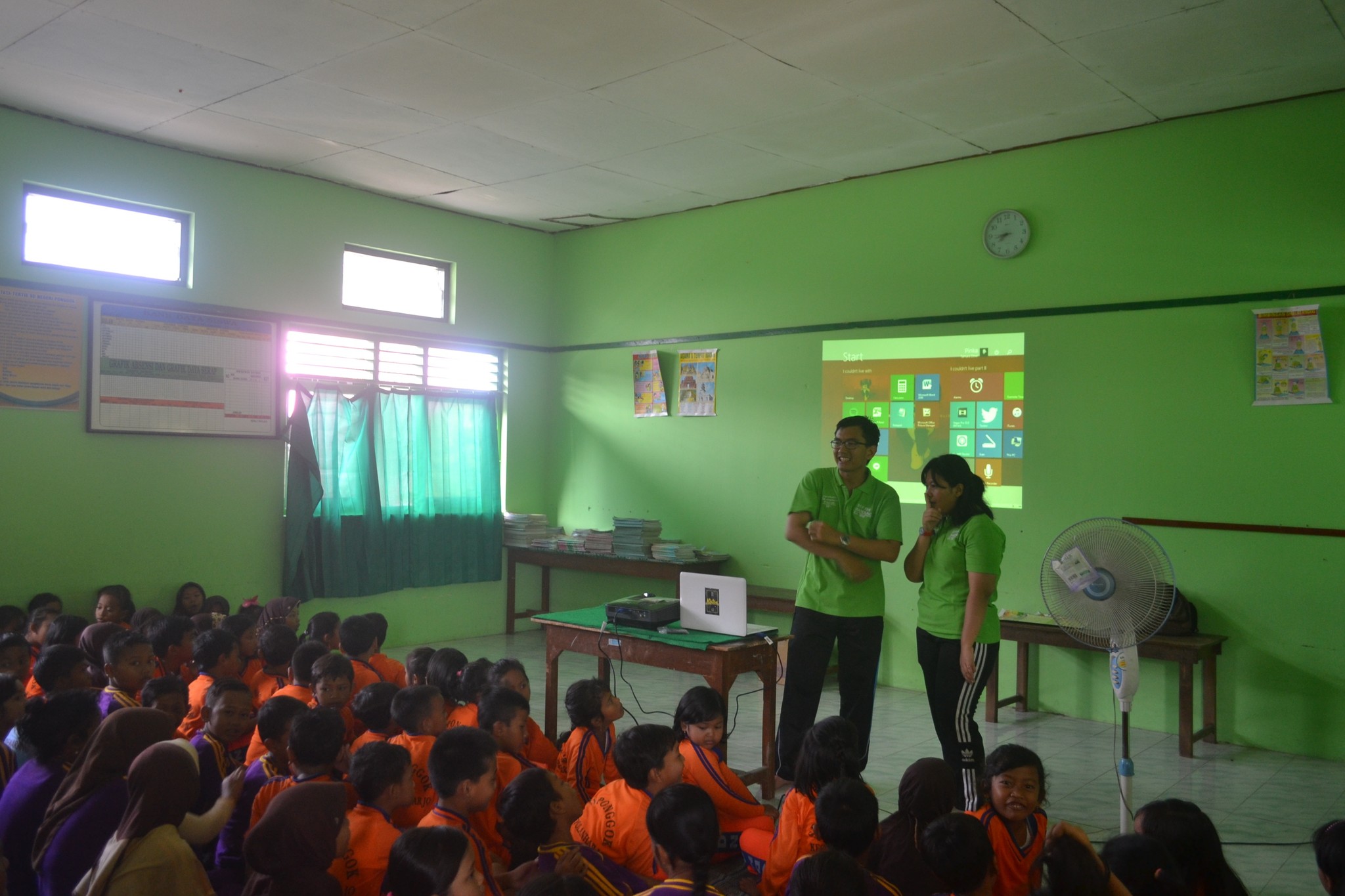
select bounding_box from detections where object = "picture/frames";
[83,297,282,439]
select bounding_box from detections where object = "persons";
[757,415,904,787]
[904,454,1006,809]
[0,578,1343,896]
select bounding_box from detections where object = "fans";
[1039,517,1176,842]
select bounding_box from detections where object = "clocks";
[982,209,1032,260]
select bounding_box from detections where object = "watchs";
[839,533,850,549]
[918,526,936,537]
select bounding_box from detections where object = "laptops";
[679,570,777,637]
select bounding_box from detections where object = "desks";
[747,584,797,617]
[524,605,779,812]
[502,545,728,636]
[982,608,1227,763]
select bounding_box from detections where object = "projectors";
[605,592,679,629]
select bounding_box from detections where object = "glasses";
[828,437,868,451]
